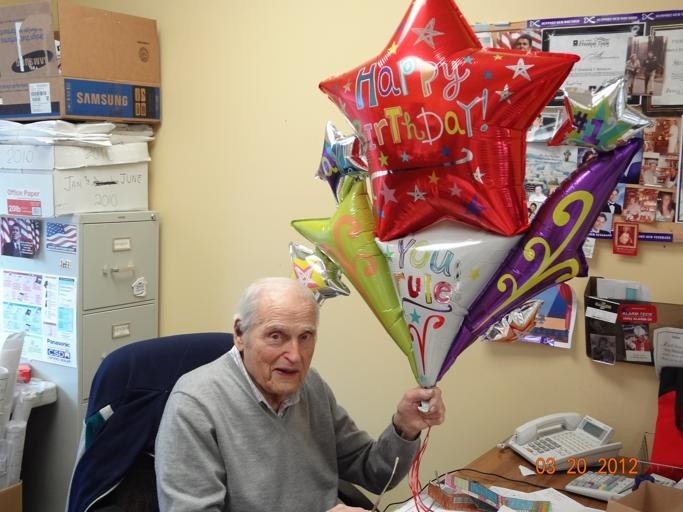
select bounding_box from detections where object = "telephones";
[495,412,624,472]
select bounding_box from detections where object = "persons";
[643,162,661,182]
[657,190,675,222]
[666,167,677,187]
[152,276,446,511]
[667,121,677,154]
[1,223,28,258]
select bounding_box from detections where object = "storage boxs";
[0,1,162,216]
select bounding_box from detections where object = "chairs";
[66,333,233,511]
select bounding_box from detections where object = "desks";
[395,435,681,512]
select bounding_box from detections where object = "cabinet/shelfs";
[1,211,159,512]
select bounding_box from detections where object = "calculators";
[564,471,636,502]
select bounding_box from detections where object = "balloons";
[286,0,655,413]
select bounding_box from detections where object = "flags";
[0,216,38,254]
[46,222,76,249]
